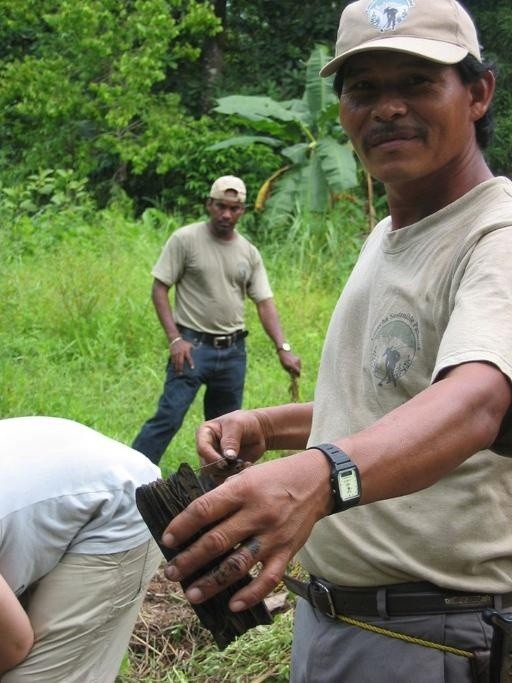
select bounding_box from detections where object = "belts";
[273,569,512,620]
[175,323,250,350]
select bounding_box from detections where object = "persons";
[131,173,301,466]
[166,0,510,680]
[0,413,164,682]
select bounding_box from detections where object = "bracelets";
[168,335,184,347]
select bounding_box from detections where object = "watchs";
[276,342,291,353]
[314,436,361,513]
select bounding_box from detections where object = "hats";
[316,0,484,79]
[208,174,247,203]
